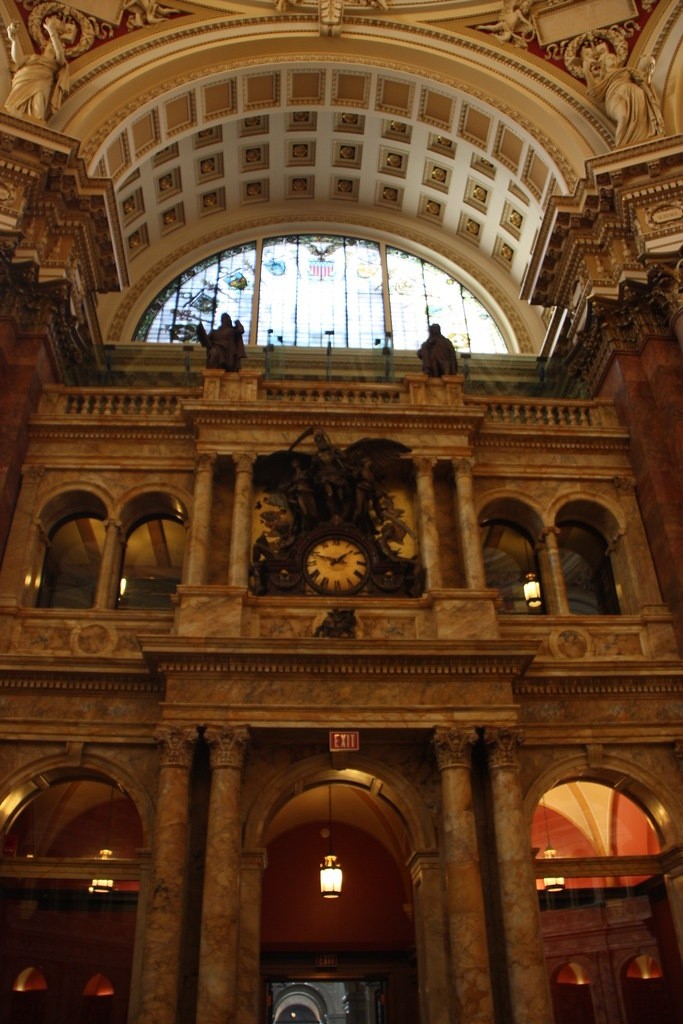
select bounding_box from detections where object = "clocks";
[304,534,373,597]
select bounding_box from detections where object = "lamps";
[91,786,118,893]
[522,542,542,610]
[319,784,343,898]
[540,796,566,892]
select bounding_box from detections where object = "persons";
[196,313,246,373]
[4,18,69,124]
[476,0,536,46]
[417,323,458,377]
[285,429,395,526]
[584,52,664,147]
